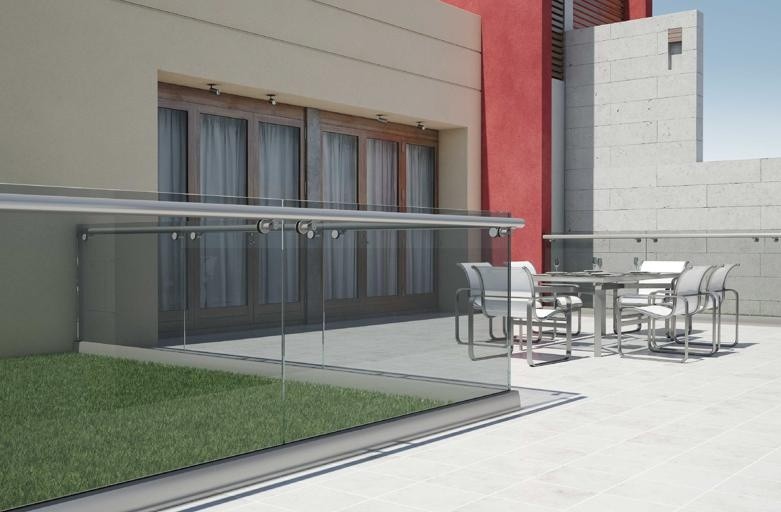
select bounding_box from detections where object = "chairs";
[653,259,742,357]
[454,259,543,350]
[615,262,719,364]
[468,260,577,366]
[501,259,585,341]
[614,257,690,340]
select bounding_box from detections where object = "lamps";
[206,84,220,96]
[376,113,389,125]
[415,121,427,133]
[266,93,277,107]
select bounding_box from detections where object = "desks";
[528,268,680,358]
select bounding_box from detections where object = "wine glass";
[552,254,560,272]
[591,256,603,270]
[634,257,645,272]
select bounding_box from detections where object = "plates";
[630,271,649,274]
[582,270,610,276]
[545,271,568,274]
